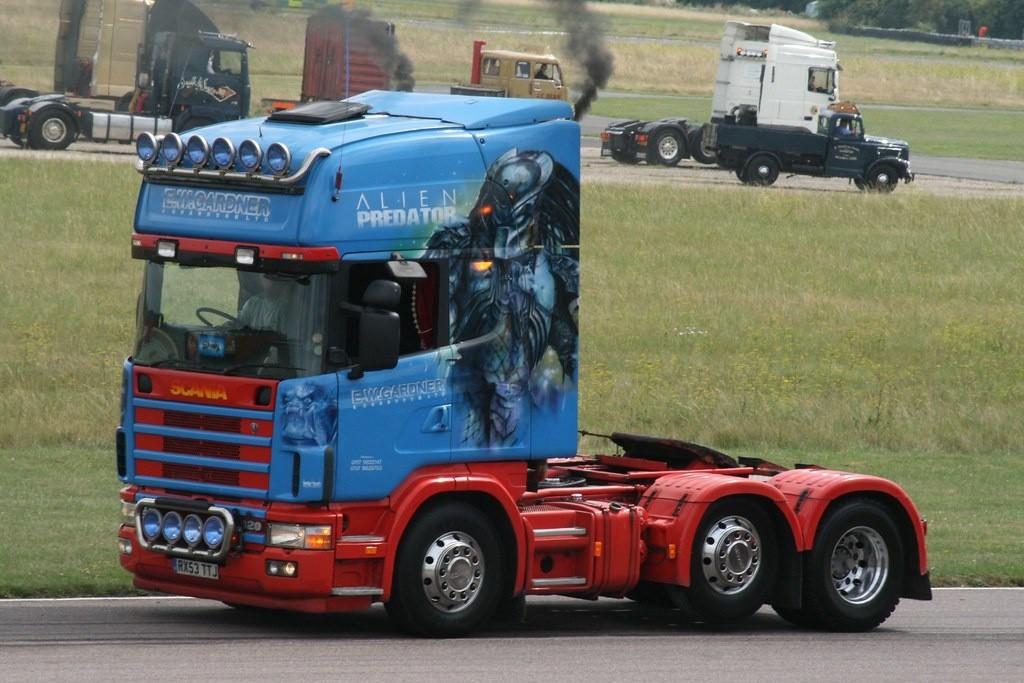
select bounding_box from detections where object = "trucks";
[261,6,395,112]
[451,40,568,104]
[0,29,257,151]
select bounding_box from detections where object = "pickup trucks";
[704,101,913,193]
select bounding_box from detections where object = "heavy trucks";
[601,19,843,168]
[115,90,934,641]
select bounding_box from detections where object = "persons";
[534,63,548,79]
[398,262,439,357]
[223,269,303,366]
[808,76,817,93]
